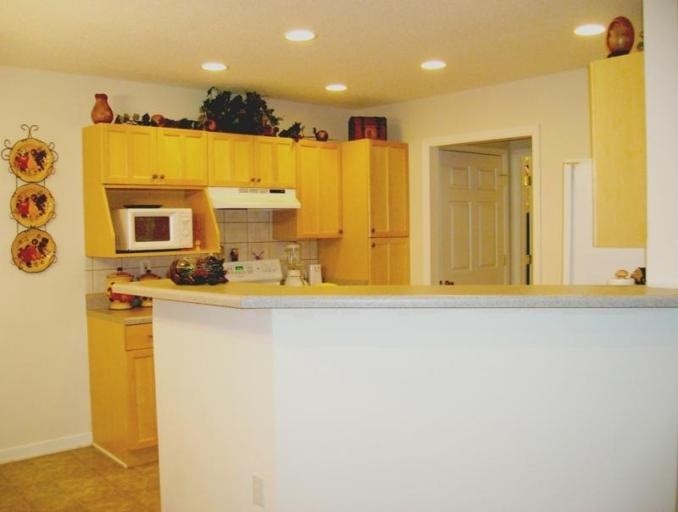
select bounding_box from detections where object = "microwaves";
[109,206,196,252]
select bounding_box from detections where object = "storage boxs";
[348,116,387,140]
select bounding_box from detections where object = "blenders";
[283,242,307,287]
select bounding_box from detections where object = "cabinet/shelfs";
[87,317,157,469]
[272,138,343,241]
[82,123,296,191]
[316,137,411,285]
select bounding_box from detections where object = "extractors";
[204,185,301,210]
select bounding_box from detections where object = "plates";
[10,137,57,273]
